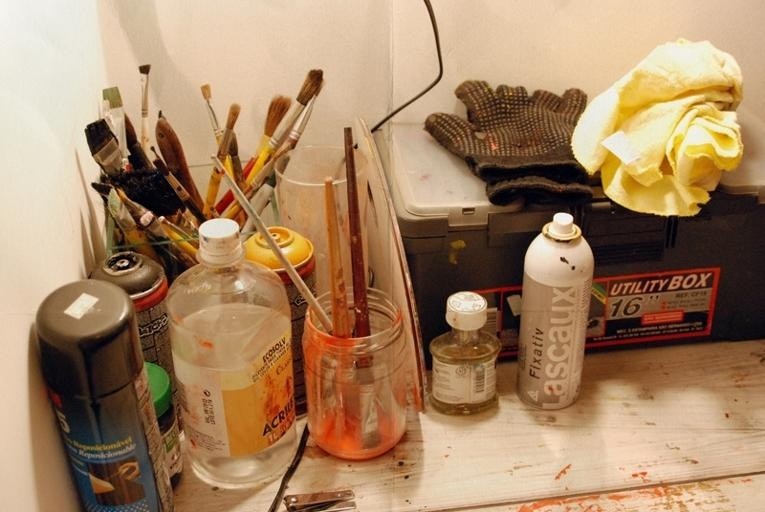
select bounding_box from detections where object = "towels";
[571,38,744,218]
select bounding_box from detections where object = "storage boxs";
[375,119,764,371]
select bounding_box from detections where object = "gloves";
[467,109,595,209]
[425,79,588,179]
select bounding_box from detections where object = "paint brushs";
[84,64,324,272]
[210,127,380,448]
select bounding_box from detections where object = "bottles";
[300,285,409,463]
[165,217,298,489]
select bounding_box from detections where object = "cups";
[272,143,370,297]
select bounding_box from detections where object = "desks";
[169,339,764,512]
